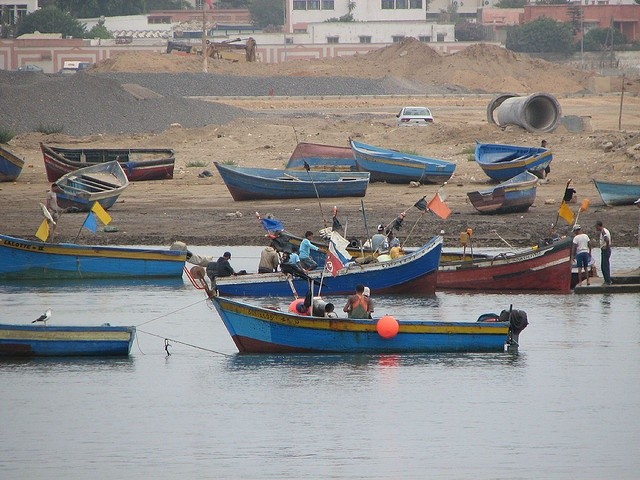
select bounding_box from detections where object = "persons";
[299,231,327,271]
[290,246,307,272]
[570,224,591,286]
[541,140,550,177]
[45,183,59,242]
[593,221,613,285]
[258,242,278,273]
[362,287,374,319]
[563,187,576,203]
[343,284,370,319]
[372,224,389,251]
[217,252,236,277]
[386,230,400,248]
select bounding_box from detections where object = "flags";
[558,201,574,224]
[329,230,353,267]
[261,218,284,231]
[428,194,451,219]
[581,198,589,211]
[83,212,97,234]
[34,219,50,241]
[91,200,112,225]
[414,196,428,211]
[325,251,342,277]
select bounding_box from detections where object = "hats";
[224,252,231,260]
[571,224,580,232]
[378,225,384,231]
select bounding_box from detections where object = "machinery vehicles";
[166,36,257,63]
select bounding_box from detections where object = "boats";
[592,177,640,207]
[204,284,527,355]
[467,169,539,214]
[0,146,24,182]
[347,136,456,187]
[39,142,176,181]
[208,229,446,298]
[0,325,136,357]
[285,140,356,169]
[51,156,130,212]
[213,162,370,201]
[0,234,188,280]
[474,137,552,182]
[255,211,595,273]
[437,236,575,290]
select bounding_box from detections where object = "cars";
[14,64,43,72]
[396,106,435,126]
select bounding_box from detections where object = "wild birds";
[31,308,54,326]
